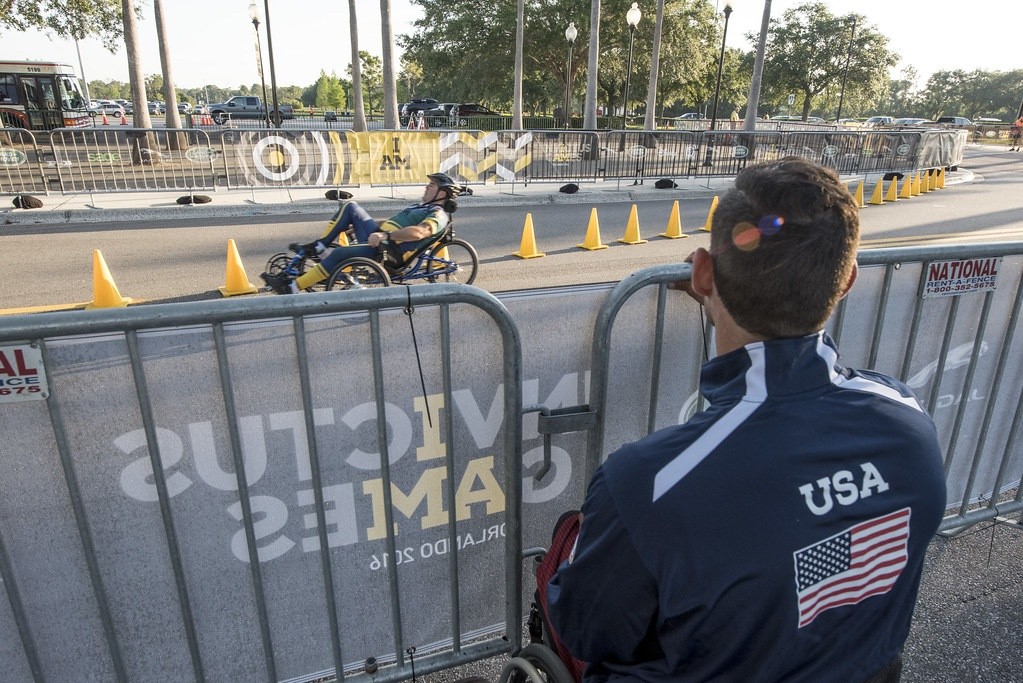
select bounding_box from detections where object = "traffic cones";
[513,212,546,260]
[100,110,129,126]
[218,239,258,297]
[854,165,946,208]
[335,231,366,284]
[421,241,450,269]
[617,206,649,244]
[577,208,609,251]
[189,111,213,125]
[84,249,131,310]
[659,193,724,239]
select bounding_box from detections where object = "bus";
[0,60,94,144]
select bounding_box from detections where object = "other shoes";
[261,272,291,294]
[1016,148,1020,151]
[1009,148,1014,151]
[288,242,319,257]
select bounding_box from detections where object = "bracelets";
[386,232,392,241]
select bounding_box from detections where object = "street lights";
[248,2,275,148]
[560,21,578,146]
[617,1,641,150]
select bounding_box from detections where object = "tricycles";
[265,198,479,325]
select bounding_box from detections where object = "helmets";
[427,173,461,199]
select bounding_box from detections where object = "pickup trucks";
[205,95,295,125]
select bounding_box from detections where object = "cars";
[675,112,1004,140]
[324,98,503,128]
[85,97,214,118]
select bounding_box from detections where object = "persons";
[310,108,314,119]
[260,172,463,295]
[1009,115,1023,151]
[546,154,947,683]
[730,106,740,130]
[763,112,769,119]
